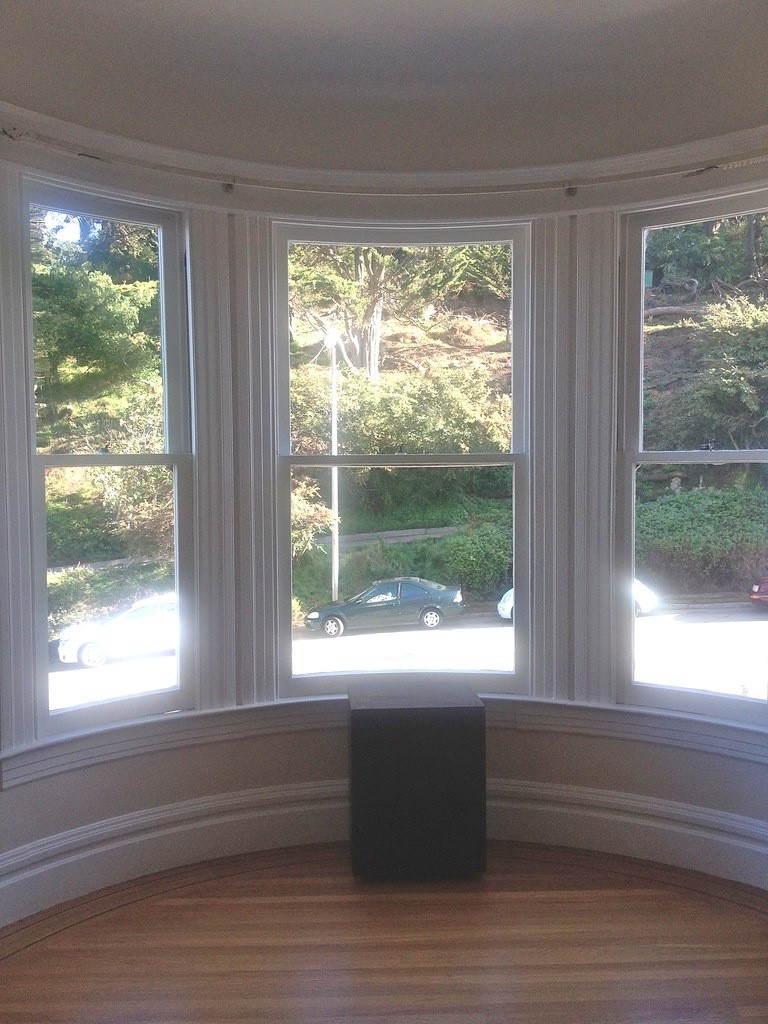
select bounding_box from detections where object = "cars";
[304,577,465,637]
[58,593,179,668]
[750,577,768,607]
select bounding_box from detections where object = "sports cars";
[498,576,662,620]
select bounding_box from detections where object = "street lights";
[322,331,343,602]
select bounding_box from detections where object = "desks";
[349,680,487,888]
